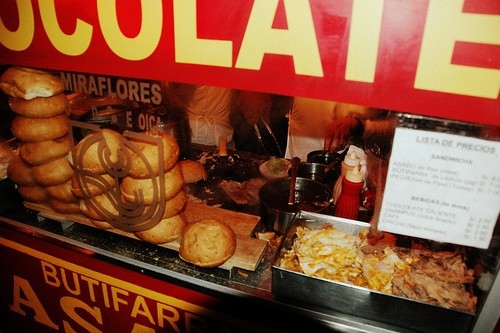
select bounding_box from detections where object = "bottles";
[332,151,360,206]
[333,164,365,219]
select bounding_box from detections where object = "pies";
[218,176,268,204]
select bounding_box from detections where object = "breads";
[0,66,236,268]
[259,157,292,180]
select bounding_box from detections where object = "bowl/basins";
[258,177,332,236]
[308,149,342,167]
[288,162,340,185]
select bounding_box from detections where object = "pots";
[61,88,190,163]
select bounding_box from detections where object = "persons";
[166,82,271,150]
[284,97,399,163]
